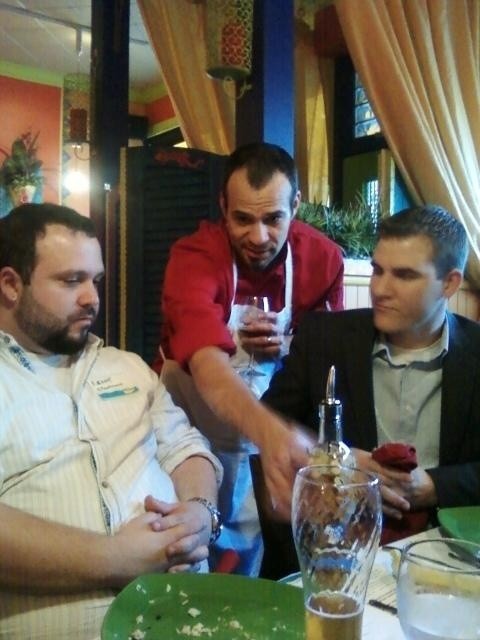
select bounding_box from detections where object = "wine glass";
[236,295,271,375]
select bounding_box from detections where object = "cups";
[291,466,479,640]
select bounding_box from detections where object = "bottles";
[308,363,357,471]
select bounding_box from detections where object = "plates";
[100,572,307,640]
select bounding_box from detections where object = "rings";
[267,334,272,345]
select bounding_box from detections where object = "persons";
[152,141,344,579]
[249,204,480,583]
[1,201,224,640]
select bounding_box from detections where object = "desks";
[266,521,479,638]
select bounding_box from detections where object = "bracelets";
[187,497,223,547]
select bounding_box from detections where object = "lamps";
[203,21,261,104]
[62,105,99,164]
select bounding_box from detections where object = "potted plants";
[4,151,42,208]
